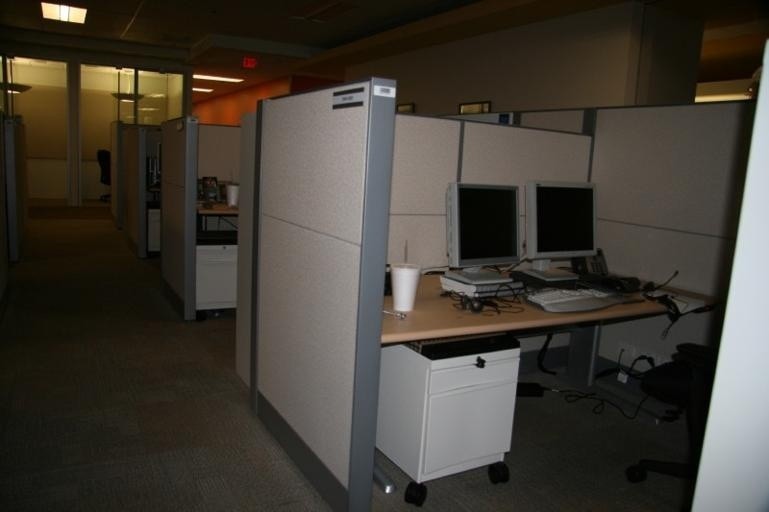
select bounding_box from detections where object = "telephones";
[573,247,609,276]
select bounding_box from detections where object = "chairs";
[625,358,718,511]
[96,150,112,203]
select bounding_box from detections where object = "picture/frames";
[395,102,415,113]
[459,101,491,115]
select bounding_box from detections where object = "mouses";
[643,290,669,300]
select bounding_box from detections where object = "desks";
[373,266,725,494]
[147,185,161,202]
[196,205,239,230]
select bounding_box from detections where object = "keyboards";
[524,286,628,312]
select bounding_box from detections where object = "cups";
[389,262,421,313]
[224,184,240,208]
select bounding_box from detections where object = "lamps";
[0,56,32,94]
[112,67,144,104]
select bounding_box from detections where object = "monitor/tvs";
[444,181,521,285]
[523,178,598,282]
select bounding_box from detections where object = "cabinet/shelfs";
[147,201,160,252]
[374,333,522,485]
[196,230,239,310]
